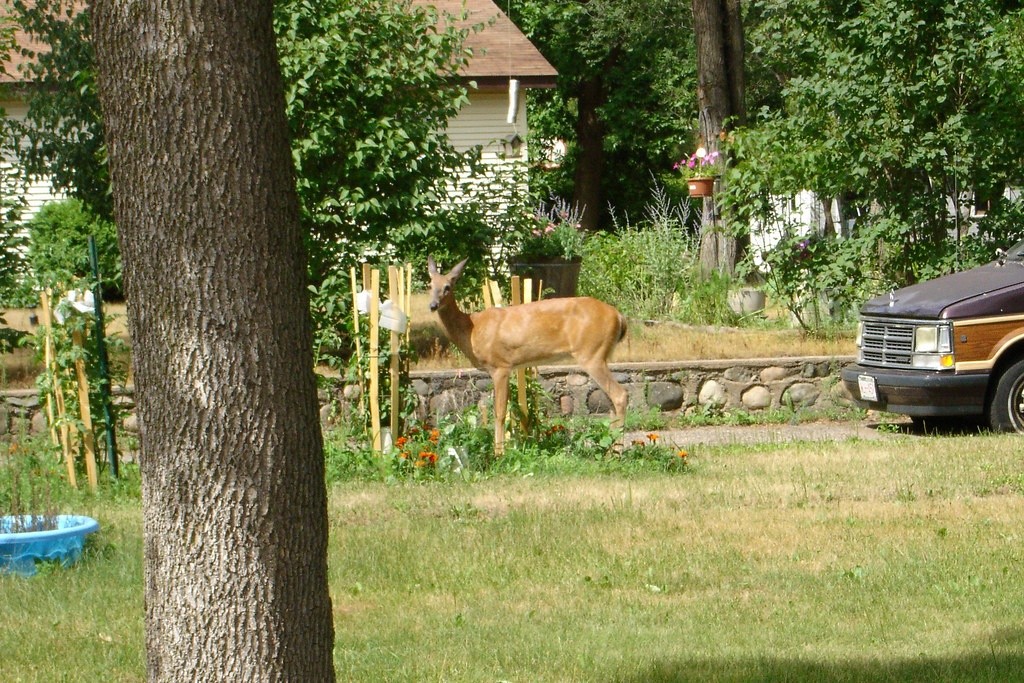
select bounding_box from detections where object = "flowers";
[673,148,723,180]
[508,207,585,263]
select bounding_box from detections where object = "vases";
[686,177,714,198]
[505,254,582,302]
[727,288,766,317]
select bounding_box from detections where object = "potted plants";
[0,409,99,575]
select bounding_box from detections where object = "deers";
[426,252,628,459]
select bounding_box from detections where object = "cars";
[840,237,1024,434]
